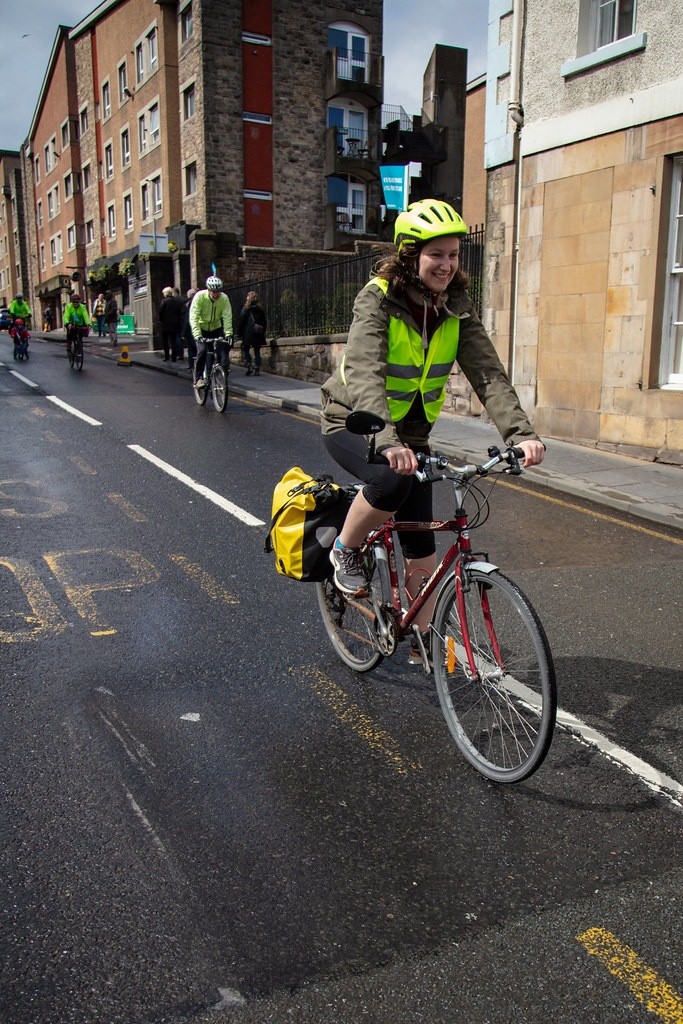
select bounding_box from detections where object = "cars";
[0,309,11,330]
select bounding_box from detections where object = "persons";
[44,303,54,332]
[10,293,32,348]
[92,290,118,347]
[188,276,234,399]
[11,319,30,360]
[320,199,544,682]
[64,293,92,361]
[238,291,268,376]
[159,286,201,369]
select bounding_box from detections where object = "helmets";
[394,199,469,254]
[15,293,23,299]
[70,294,80,300]
[15,319,24,325]
[206,276,223,291]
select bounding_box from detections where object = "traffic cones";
[117,345,133,366]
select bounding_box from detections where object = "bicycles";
[312,412,559,787]
[192,337,232,413]
[67,321,90,371]
[9,314,30,338]
[13,335,29,360]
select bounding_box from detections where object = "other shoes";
[254,368,259,376]
[246,367,252,377]
[162,354,195,369]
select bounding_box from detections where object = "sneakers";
[330,537,374,595]
[196,379,206,388]
[408,639,435,668]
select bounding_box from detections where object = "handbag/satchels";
[265,467,350,582]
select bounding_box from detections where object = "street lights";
[66,266,88,314]
